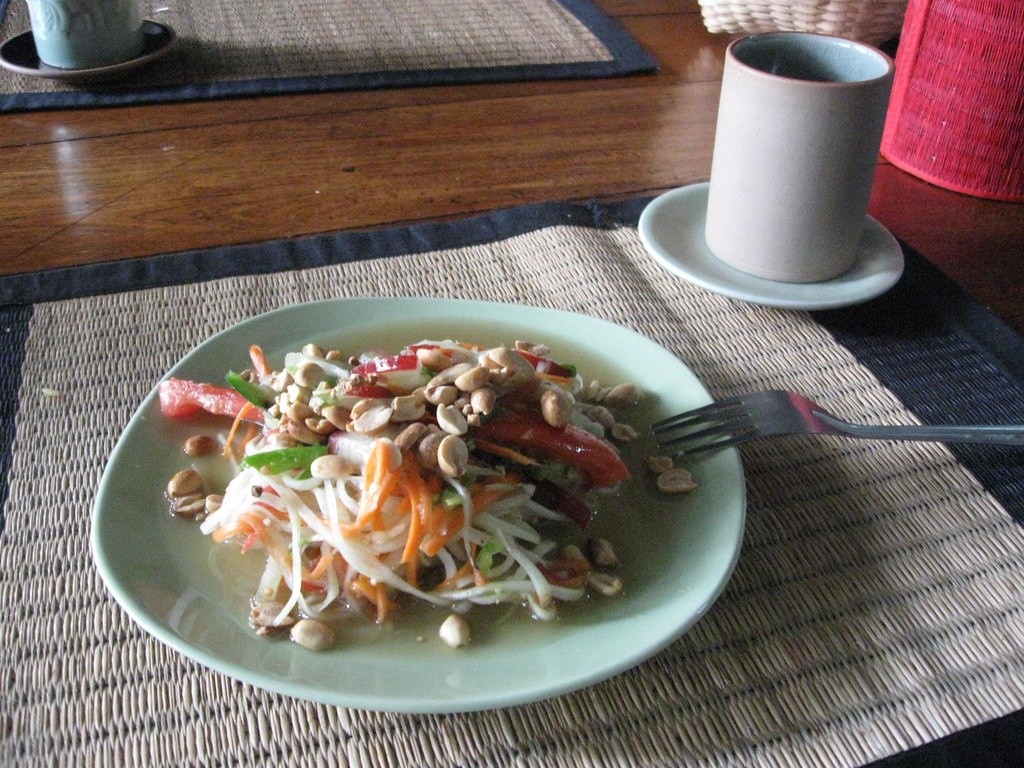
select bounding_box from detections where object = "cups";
[705,33,895,283]
[26,0,145,69]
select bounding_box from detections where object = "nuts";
[166,344,696,651]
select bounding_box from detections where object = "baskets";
[698,0,910,49]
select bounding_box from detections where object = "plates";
[89,298,749,715]
[0,19,176,83]
[637,181,903,310]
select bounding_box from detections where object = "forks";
[650,390,1023,461]
